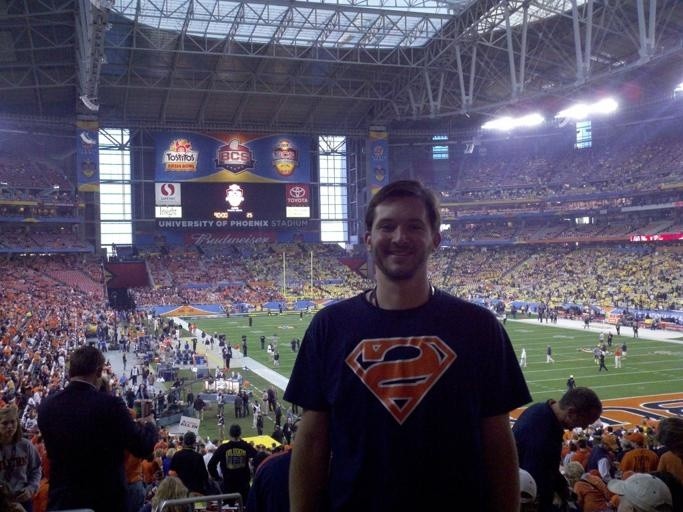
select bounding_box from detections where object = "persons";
[511,385,682,511]
[592,332,628,370]
[259,334,280,366]
[520,347,528,367]
[416,139,683,333]
[567,375,576,392]
[283,179,533,511]
[0,249,212,511]
[210,366,302,511]
[249,317,253,326]
[0,158,91,251]
[210,330,233,368]
[289,337,300,351]
[546,344,554,364]
[121,242,377,318]
[241,335,249,356]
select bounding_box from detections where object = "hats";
[625,434,643,444]
[608,472,673,511]
[518,469,537,502]
[605,435,618,449]
[184,432,196,444]
